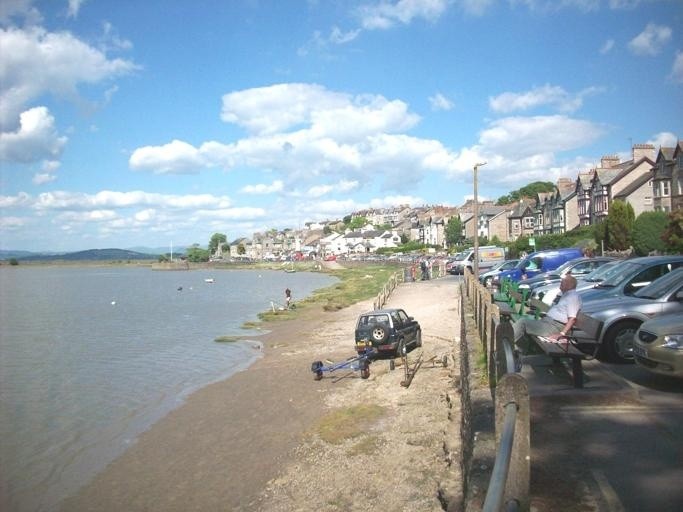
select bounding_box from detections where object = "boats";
[205,278,214,284]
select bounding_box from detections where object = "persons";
[286,288,291,301]
[511,270,583,344]
[584,248,592,257]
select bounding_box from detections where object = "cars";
[210,245,505,275]
[479,247,683,380]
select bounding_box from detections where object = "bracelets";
[560,331,566,336]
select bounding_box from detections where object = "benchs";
[487,277,604,390]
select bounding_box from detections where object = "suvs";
[352,309,422,358]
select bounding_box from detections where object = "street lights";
[472,160,489,283]
